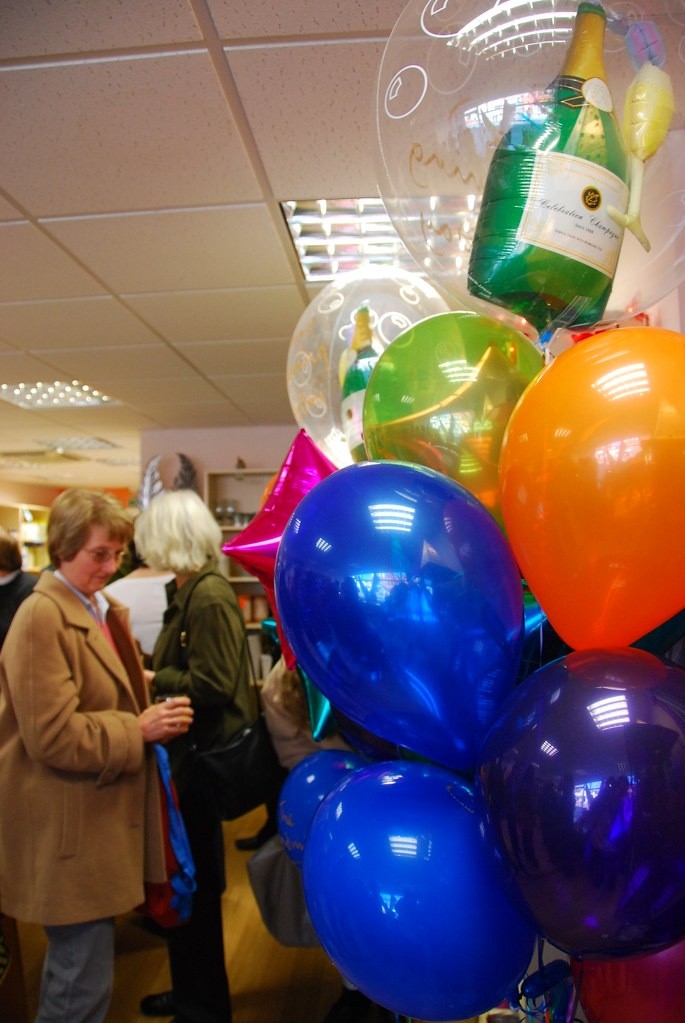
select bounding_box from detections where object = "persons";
[0,487,195,1023]
[0,527,40,651]
[133,488,406,1023]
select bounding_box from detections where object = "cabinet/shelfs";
[204,468,281,686]
[0,503,50,575]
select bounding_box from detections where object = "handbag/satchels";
[174,721,274,822]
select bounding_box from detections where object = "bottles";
[465,1,629,326]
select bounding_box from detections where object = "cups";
[156,695,188,729]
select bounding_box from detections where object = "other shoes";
[233,803,279,850]
[141,990,180,1017]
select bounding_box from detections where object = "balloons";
[220,429,338,670]
[277,750,538,1023]
[362,310,543,526]
[478,646,685,961]
[498,327,685,651]
[274,460,524,770]
[286,264,467,470]
[376,0,685,368]
[568,942,685,1023]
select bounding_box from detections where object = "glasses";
[78,545,127,562]
